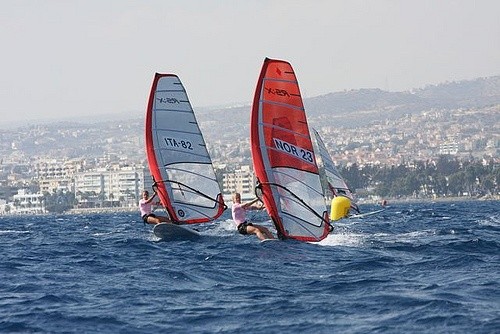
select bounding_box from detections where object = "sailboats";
[315,127,385,220]
[250,57,332,242]
[145,72,226,240]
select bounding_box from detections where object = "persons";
[139,190,171,225]
[232,193,275,240]
